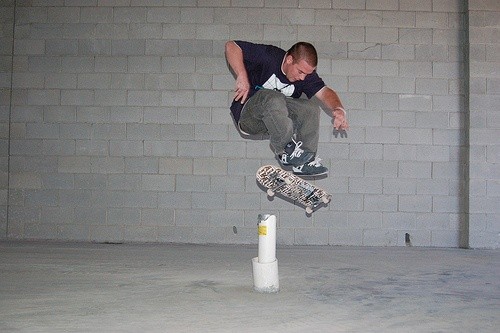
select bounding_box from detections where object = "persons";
[225,39,348,176]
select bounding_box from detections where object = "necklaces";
[275,59,292,93]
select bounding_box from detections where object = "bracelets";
[334,106,347,115]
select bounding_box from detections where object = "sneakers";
[278,141,316,167]
[291,160,330,177]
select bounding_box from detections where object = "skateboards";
[254,164,332,217]
